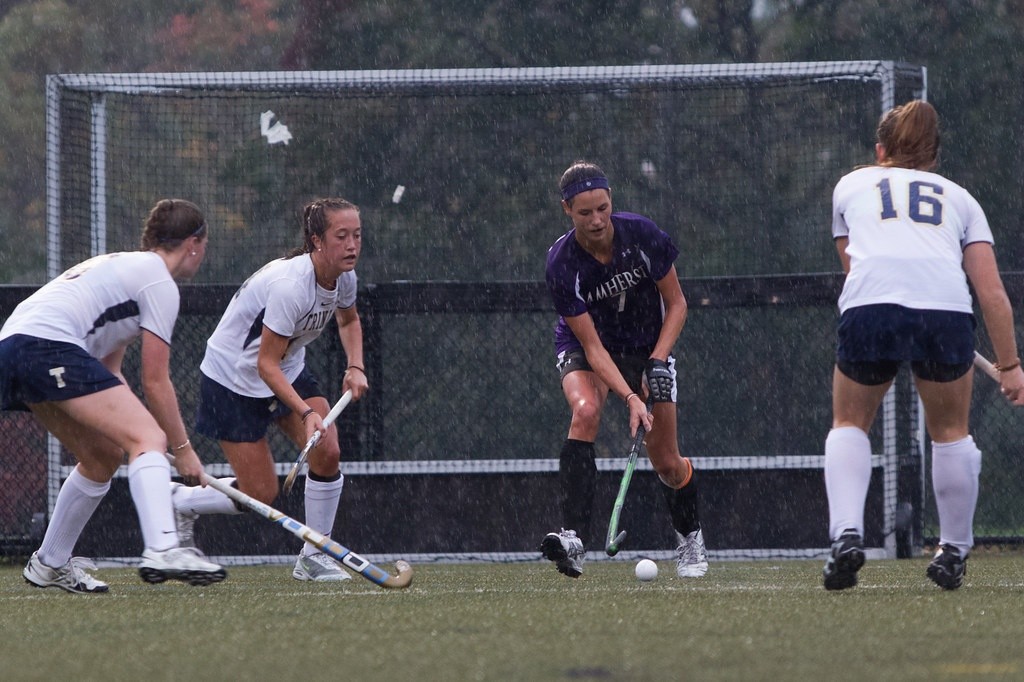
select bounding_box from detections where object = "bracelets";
[172,439,189,449]
[996,359,1020,372]
[626,393,638,405]
[349,366,364,372]
[302,409,315,423]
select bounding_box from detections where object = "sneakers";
[822,527,866,590]
[137,546,227,586]
[23,550,110,595]
[675,522,708,578]
[925,542,970,590]
[540,527,587,578]
[170,482,200,549]
[292,548,352,582]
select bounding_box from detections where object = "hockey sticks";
[607,393,655,557]
[974,349,1002,384]
[164,452,416,590]
[283,387,354,498]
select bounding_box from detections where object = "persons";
[823,100,1024,589]
[0,198,227,594]
[170,198,368,582]
[545,164,707,578]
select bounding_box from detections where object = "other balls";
[635,559,659,582]
